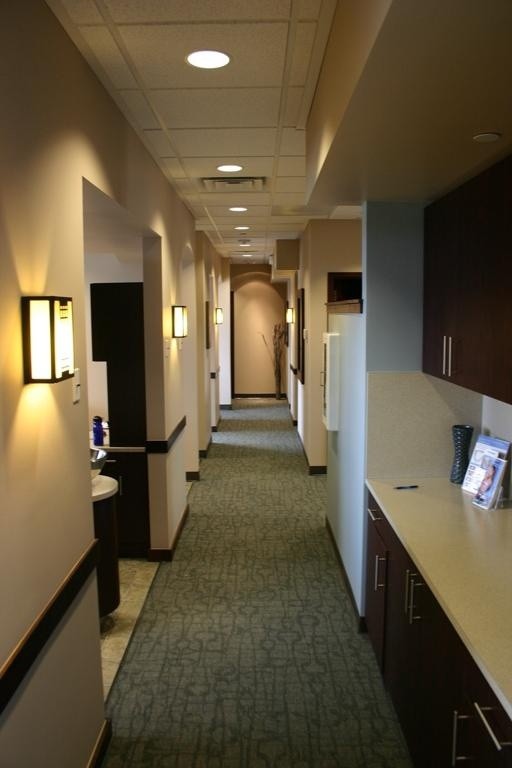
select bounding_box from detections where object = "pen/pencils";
[393,485,419,488]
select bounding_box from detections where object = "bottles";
[92,415,103,447]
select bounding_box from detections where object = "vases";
[275,369,281,400]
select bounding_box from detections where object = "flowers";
[255,322,285,369]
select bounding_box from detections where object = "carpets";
[97,555,164,704]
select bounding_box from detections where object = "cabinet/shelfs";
[365,487,388,676]
[441,609,512,768]
[422,149,512,404]
[388,521,441,768]
[87,280,147,448]
[90,448,151,560]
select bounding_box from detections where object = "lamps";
[286,306,293,323]
[17,294,75,385]
[215,307,224,324]
[171,305,188,338]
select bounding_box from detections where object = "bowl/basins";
[91,448,108,479]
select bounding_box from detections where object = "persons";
[476,465,495,501]
